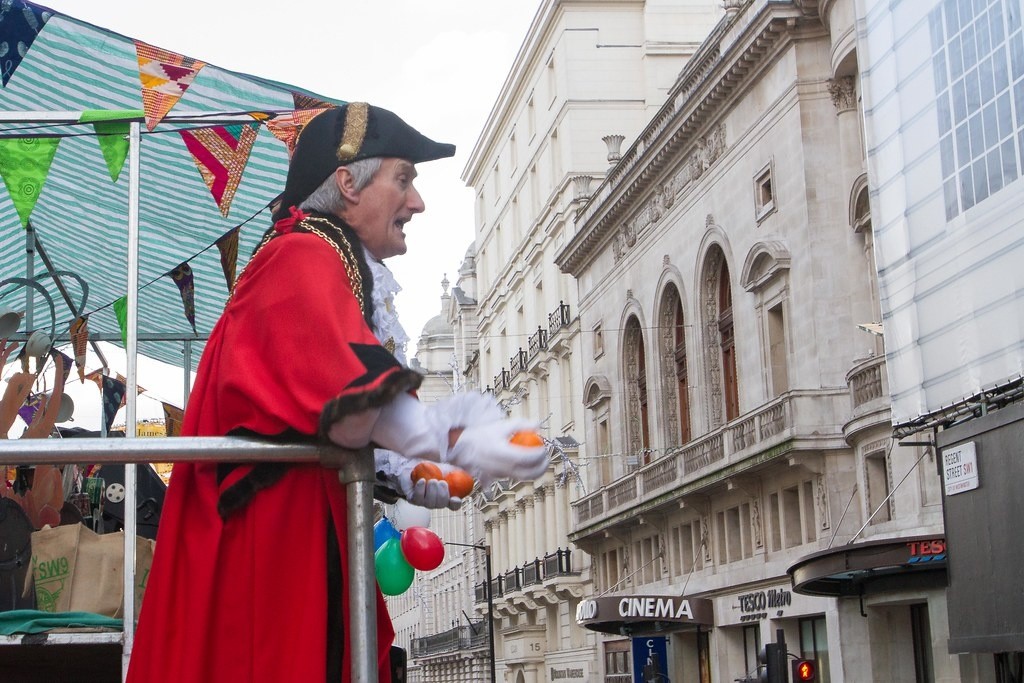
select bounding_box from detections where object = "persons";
[123,101,550,683]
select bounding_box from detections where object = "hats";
[270,100,456,222]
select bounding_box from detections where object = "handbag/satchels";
[22,521,157,633]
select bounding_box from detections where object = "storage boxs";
[82,477,105,513]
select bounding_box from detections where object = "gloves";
[391,459,462,512]
[449,421,549,482]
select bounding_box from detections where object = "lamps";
[619,623,629,637]
[655,621,664,632]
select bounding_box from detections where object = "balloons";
[401,503,431,529]
[398,526,445,571]
[373,515,403,553]
[374,534,415,596]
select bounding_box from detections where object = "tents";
[1,1,353,683]
